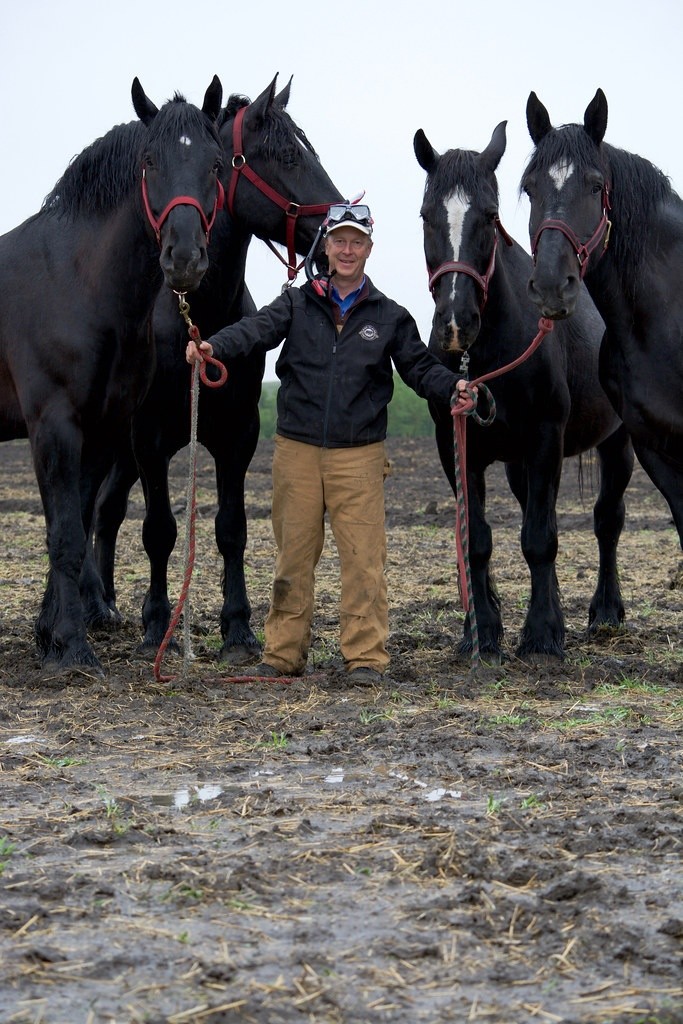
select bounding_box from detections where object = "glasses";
[327,204,371,226]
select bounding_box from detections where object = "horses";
[0,72,349,668]
[413,86,683,667]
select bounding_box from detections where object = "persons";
[186,204,479,688]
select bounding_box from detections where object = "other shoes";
[348,667,382,687]
[242,663,282,678]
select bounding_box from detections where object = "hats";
[326,220,372,235]
